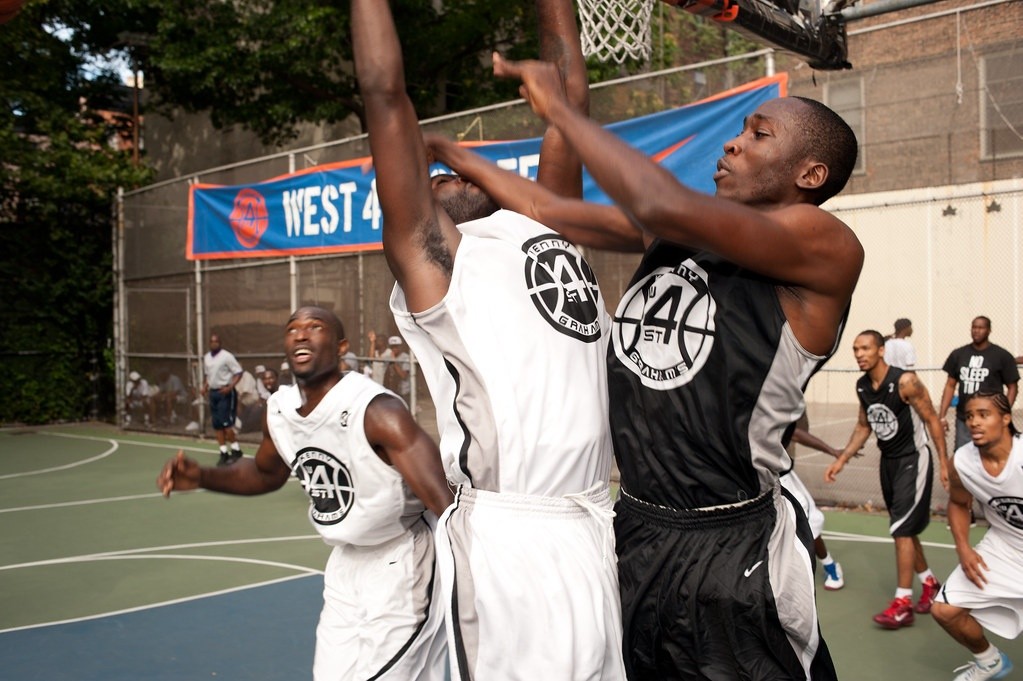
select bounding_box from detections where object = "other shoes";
[184,421,199,431]
[144,421,155,427]
[125,421,137,426]
[227,450,242,464]
[216,451,229,467]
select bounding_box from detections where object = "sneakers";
[915,575,941,614]
[875,595,915,628]
[824,562,844,590]
[953,648,1014,681]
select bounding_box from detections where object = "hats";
[255,365,266,374]
[389,336,402,345]
[894,319,911,333]
[130,371,142,381]
[280,362,290,371]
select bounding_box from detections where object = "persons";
[421,53,864,681]
[777,316,1023,681]
[123,332,409,465]
[154,307,458,681]
[348,0,627,681]
